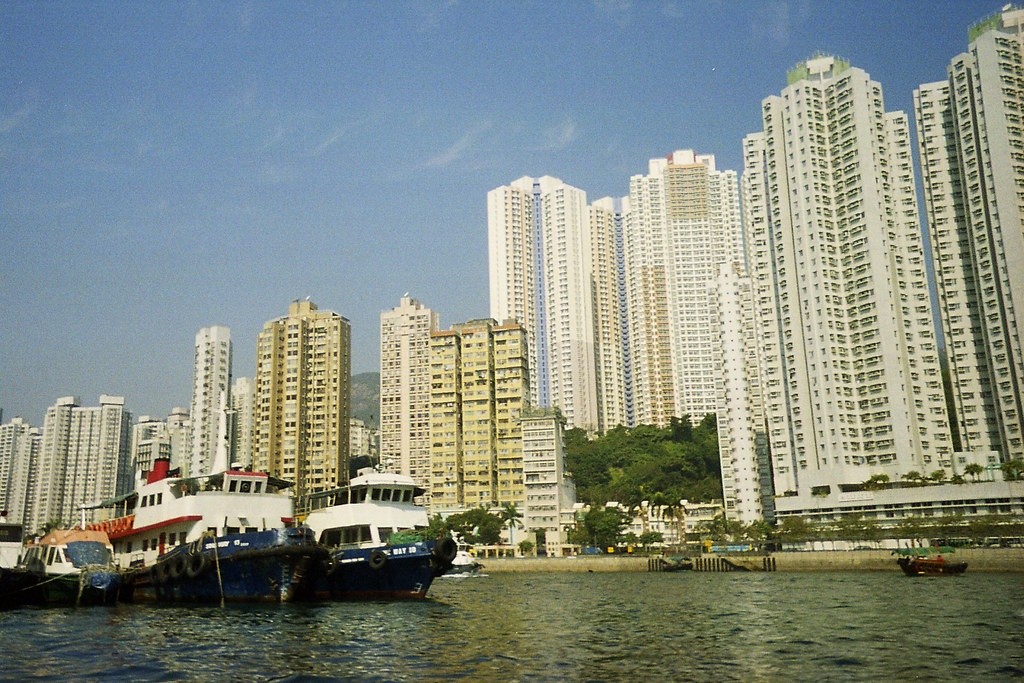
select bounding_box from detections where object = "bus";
[709,544,758,553]
[983,535,1024,549]
[930,537,974,547]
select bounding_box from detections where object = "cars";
[581,547,603,554]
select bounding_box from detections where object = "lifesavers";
[159,563,169,583]
[187,553,209,578]
[368,549,387,568]
[436,538,458,562]
[168,555,187,577]
[150,565,159,585]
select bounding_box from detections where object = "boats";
[76,456,331,607]
[897,556,968,576]
[11,525,116,577]
[440,550,485,577]
[293,455,440,604]
